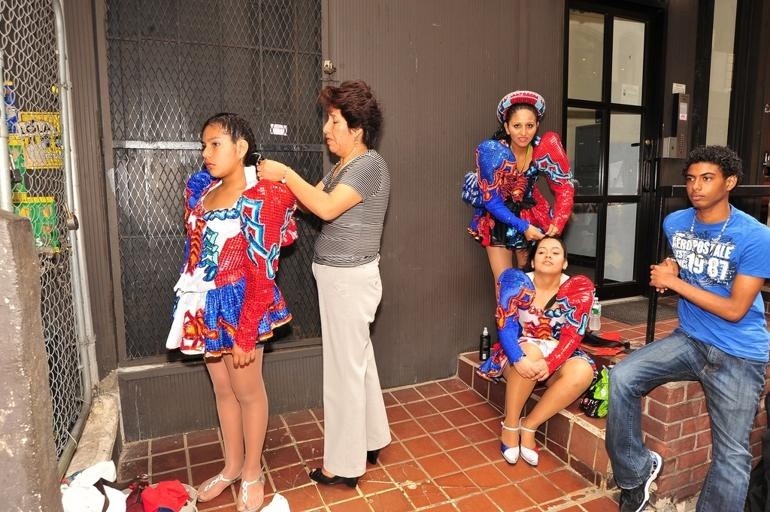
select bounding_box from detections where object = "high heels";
[308,465,359,489]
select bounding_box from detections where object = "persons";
[463,90,575,288]
[477,236,593,465]
[609,148,770,512]
[256,80,392,488]
[167,113,295,511]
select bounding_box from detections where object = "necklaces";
[690,216,731,260]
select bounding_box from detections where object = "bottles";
[589,297,602,332]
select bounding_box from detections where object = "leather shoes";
[519,417,540,467]
[500,419,520,466]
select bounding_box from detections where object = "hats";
[497,89,547,123]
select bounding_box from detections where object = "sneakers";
[348,434,381,466]
[618,448,664,512]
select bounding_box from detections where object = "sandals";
[236,462,266,512]
[196,463,248,503]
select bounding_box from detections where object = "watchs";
[281,166,288,185]
